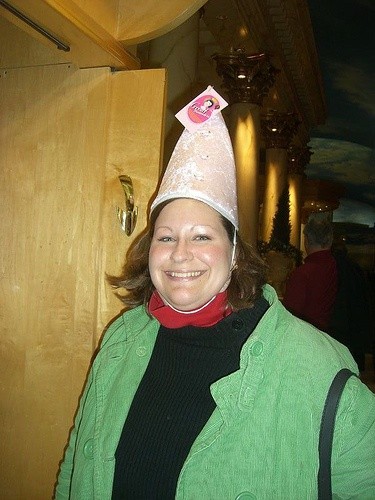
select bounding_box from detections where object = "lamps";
[260,108,300,151]
[210,45,282,107]
[286,138,316,174]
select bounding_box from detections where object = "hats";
[148,86,239,233]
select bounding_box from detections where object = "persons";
[281,213,340,330]
[53,87,375,499]
